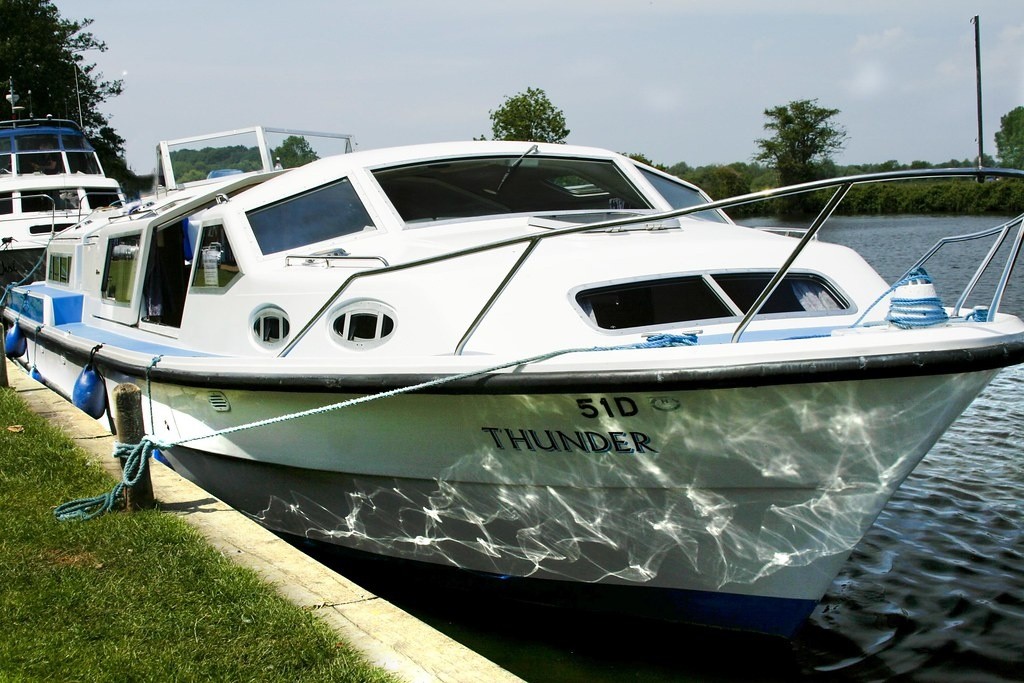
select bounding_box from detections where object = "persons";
[21,142,73,212]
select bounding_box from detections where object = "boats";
[0,123,1024,683]
[0,77,130,307]
